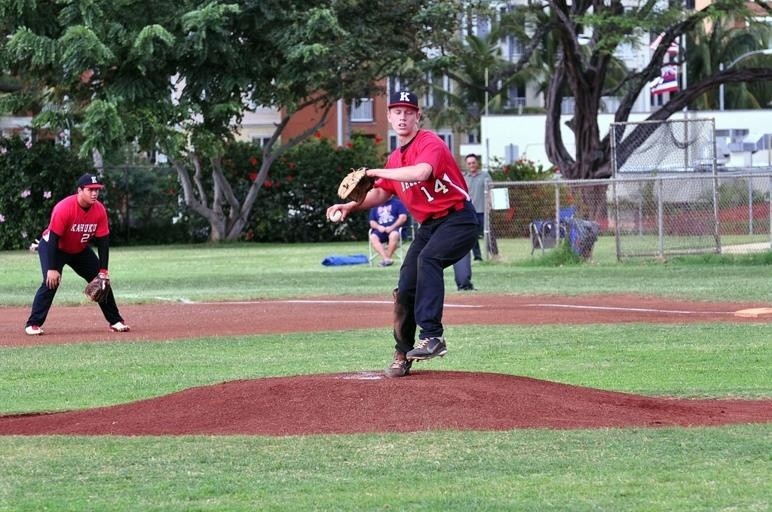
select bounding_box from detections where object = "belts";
[432,201,465,220]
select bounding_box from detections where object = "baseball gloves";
[82,270,110,303]
[337,167,378,207]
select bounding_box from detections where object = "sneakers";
[473,254,503,262]
[25,325,44,335]
[406,334,448,362]
[388,347,415,377]
[109,320,130,332]
[379,259,393,267]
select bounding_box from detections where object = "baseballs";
[329,209,342,223]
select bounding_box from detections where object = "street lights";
[720,48,772,110]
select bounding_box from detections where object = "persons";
[25,173,130,336]
[327,92,479,378]
[455,252,473,290]
[465,154,499,262]
[369,195,408,267]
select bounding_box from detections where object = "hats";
[388,90,419,110]
[78,173,104,189]
